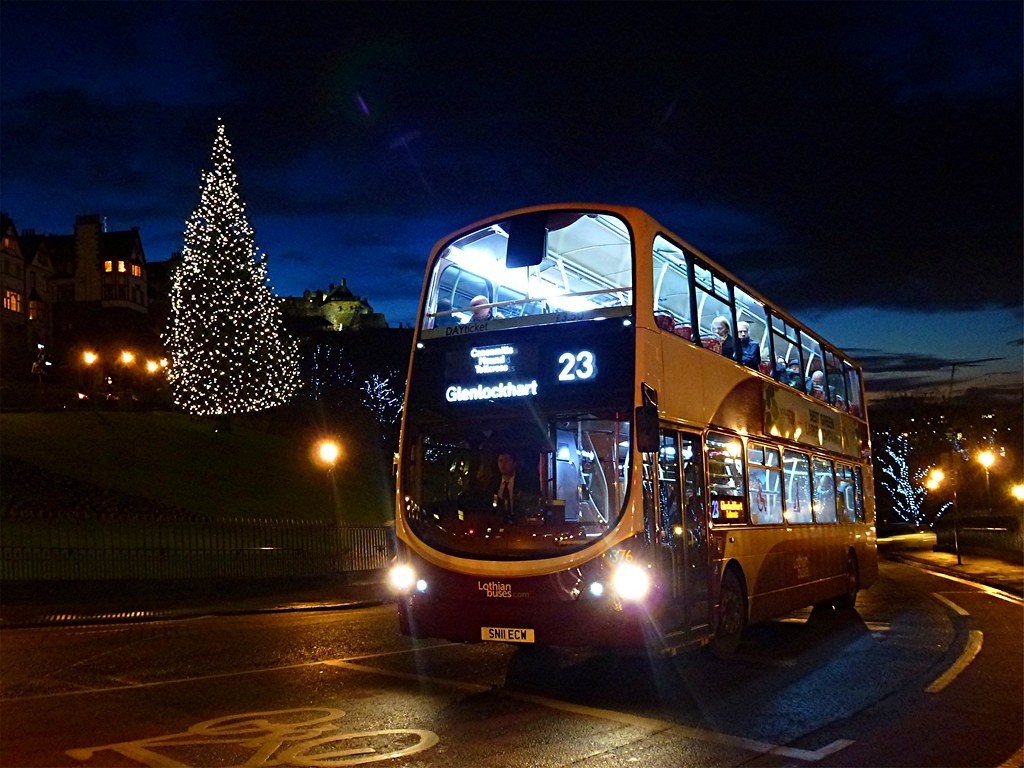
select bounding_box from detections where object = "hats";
[788,360,799,367]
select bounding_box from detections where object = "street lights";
[317,438,349,573]
[977,451,995,513]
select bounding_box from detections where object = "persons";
[789,360,813,396]
[737,322,761,372]
[770,362,788,386]
[811,370,836,407]
[475,450,536,523]
[434,295,506,327]
[815,475,837,523]
[711,317,735,359]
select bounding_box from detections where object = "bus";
[387,201,880,666]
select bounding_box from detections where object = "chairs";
[655,310,860,418]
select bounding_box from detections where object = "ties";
[502,480,511,513]
